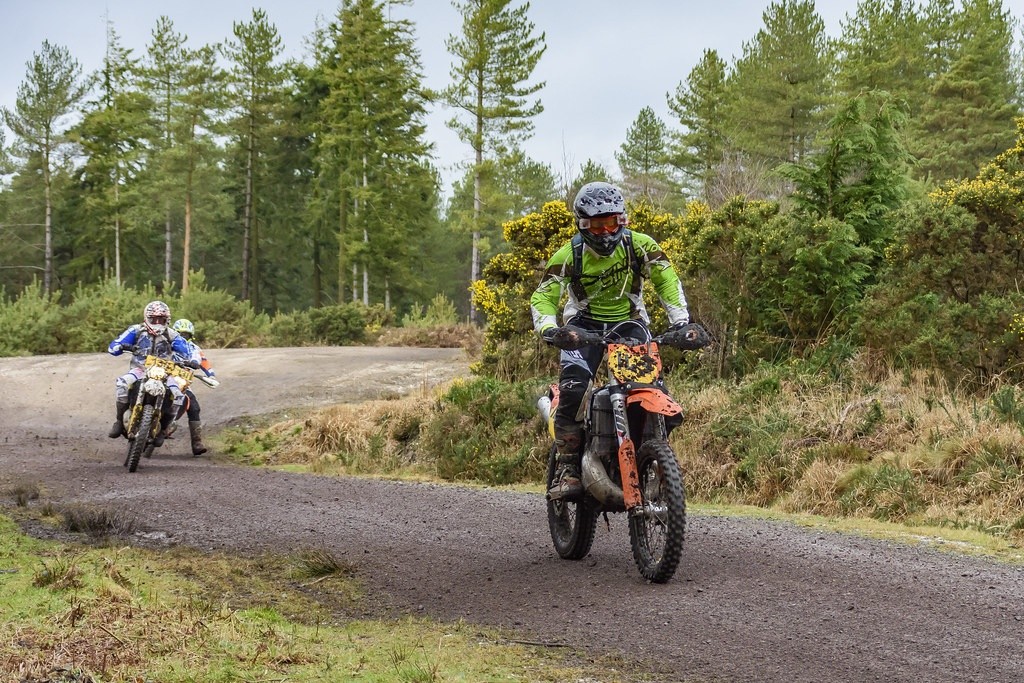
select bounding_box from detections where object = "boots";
[553,421,586,499]
[152,411,176,447]
[188,420,207,456]
[108,401,131,438]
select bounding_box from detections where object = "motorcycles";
[118,344,219,473]
[539,323,709,584]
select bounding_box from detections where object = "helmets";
[144,301,171,336]
[573,181,629,256]
[172,319,196,343]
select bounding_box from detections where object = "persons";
[172,318,216,458]
[530,181,691,502]
[107,300,201,447]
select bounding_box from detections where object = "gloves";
[189,360,200,370]
[542,327,561,346]
[669,322,688,332]
[120,343,132,351]
